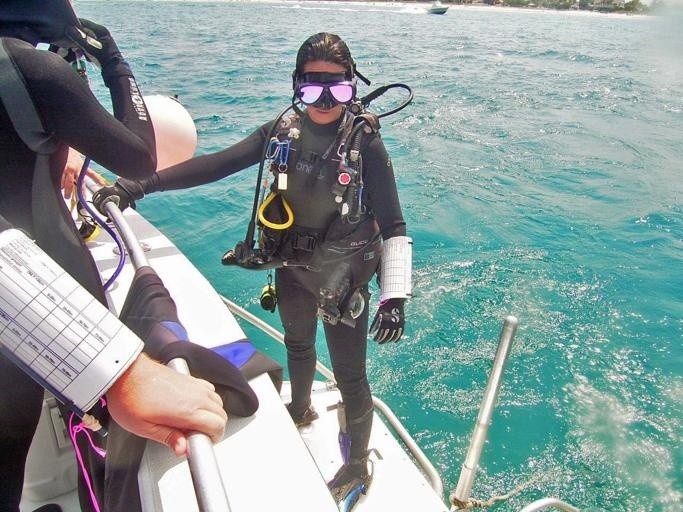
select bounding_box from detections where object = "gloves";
[48,41,85,66]
[65,17,125,69]
[92,180,135,224]
[368,299,405,345]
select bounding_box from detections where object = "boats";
[428,2,450,15]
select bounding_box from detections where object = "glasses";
[296,80,358,108]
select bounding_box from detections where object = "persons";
[47,38,88,202]
[1,213,230,457]
[1,0,159,512]
[93,33,414,512]
